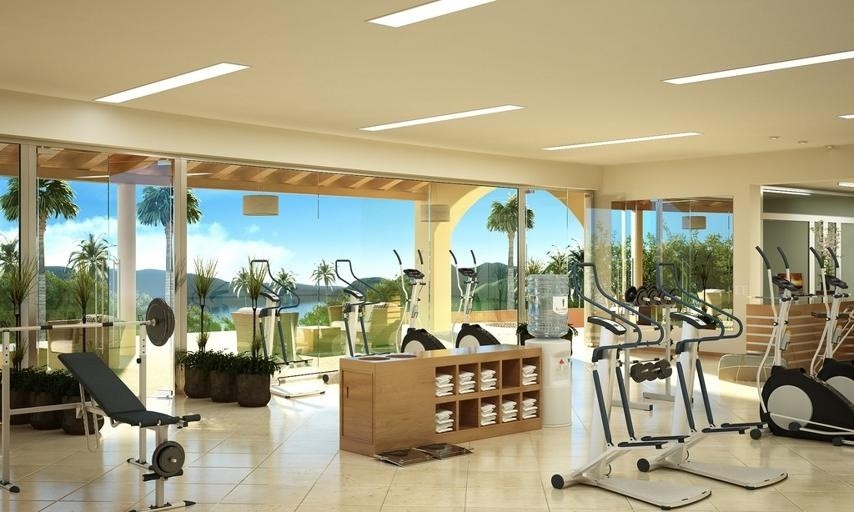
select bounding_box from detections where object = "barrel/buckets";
[526,272,571,338]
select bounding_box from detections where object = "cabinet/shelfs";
[338,343,544,456]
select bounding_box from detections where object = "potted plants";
[174,348,282,407]
[0,366,104,436]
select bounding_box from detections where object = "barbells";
[2,300,178,345]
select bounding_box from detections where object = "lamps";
[680,201,707,231]
[418,182,451,223]
[242,168,280,217]
